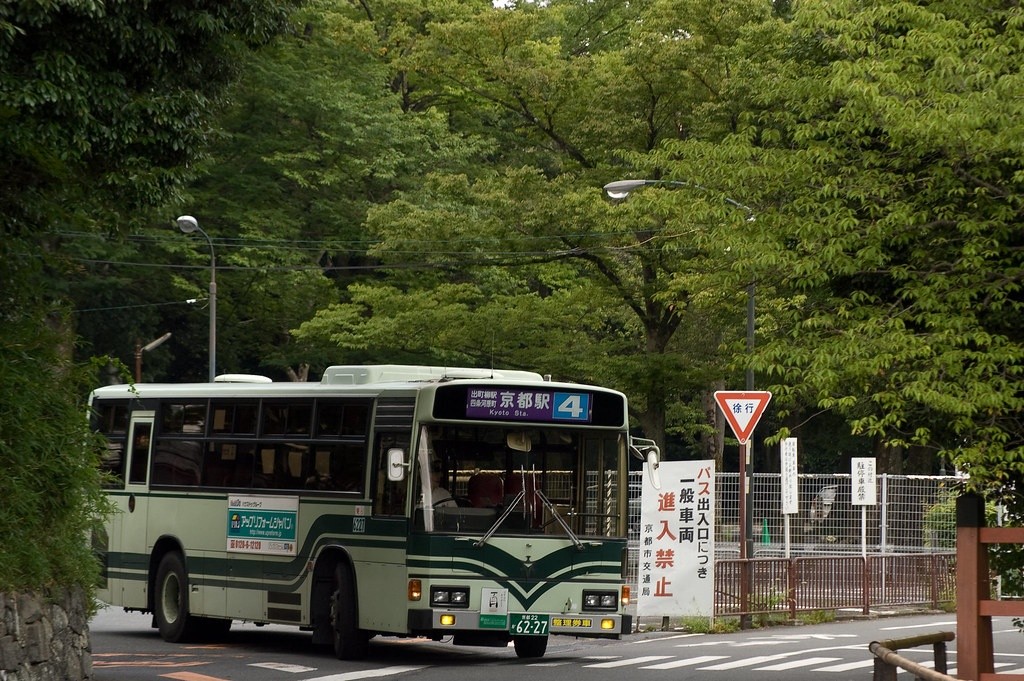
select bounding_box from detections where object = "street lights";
[137,333,171,385]
[605,178,756,610]
[177,216,217,384]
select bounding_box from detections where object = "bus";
[75,364,663,660]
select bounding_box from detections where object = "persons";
[133,431,151,482]
[416,460,458,507]
